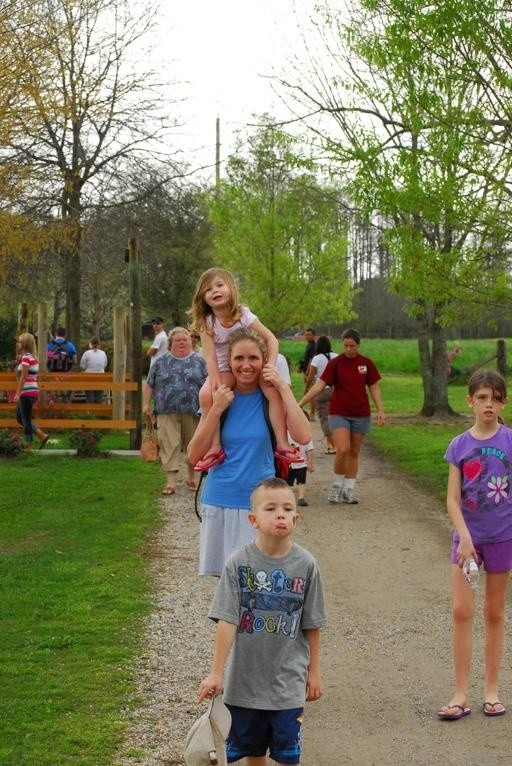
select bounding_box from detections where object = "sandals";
[193,447,225,471]
[274,447,304,463]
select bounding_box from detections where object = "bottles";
[464,556,481,590]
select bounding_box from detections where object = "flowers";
[486,475,509,503]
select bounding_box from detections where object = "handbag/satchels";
[140,415,158,461]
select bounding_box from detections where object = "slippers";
[186,480,197,490]
[161,487,175,495]
[483,702,505,715]
[438,705,471,719]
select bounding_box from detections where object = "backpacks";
[47,340,73,372]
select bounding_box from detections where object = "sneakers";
[341,488,359,503]
[327,485,342,503]
[298,499,308,505]
[39,434,49,448]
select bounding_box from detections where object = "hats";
[183,694,232,765]
[152,316,163,322]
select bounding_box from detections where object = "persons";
[143,326,208,495]
[433,369,512,719]
[187,329,203,359]
[284,407,316,506]
[47,326,78,404]
[144,316,169,427]
[195,478,325,765]
[184,325,312,579]
[12,332,49,453]
[192,266,306,472]
[302,335,340,454]
[79,335,108,403]
[298,327,385,504]
[296,328,318,421]
